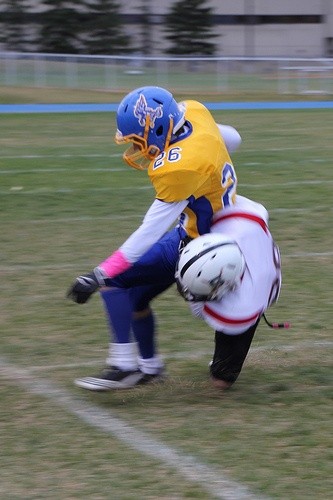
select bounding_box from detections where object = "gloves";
[65,273,100,304]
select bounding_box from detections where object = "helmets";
[175,233,245,302]
[114,86,185,172]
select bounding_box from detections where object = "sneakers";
[74,366,150,391]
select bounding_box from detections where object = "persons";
[64,84,242,392]
[174,193,283,397]
